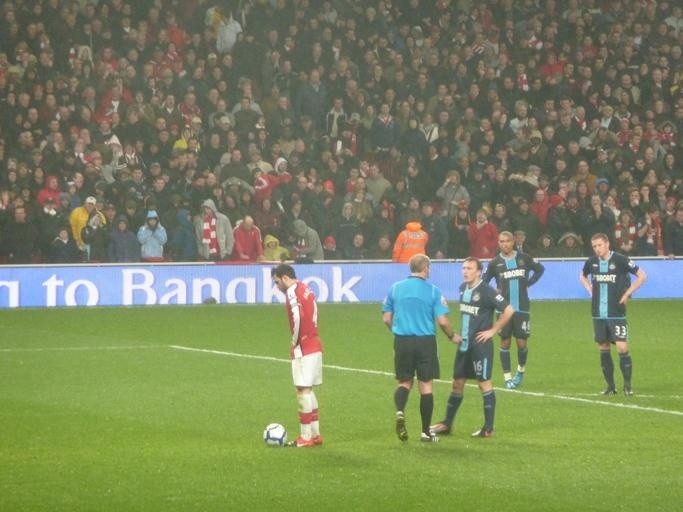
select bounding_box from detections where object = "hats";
[208,53,217,59]
[221,116,230,124]
[326,236,336,244]
[86,196,97,206]
[293,219,307,236]
[596,177,609,187]
[192,117,202,124]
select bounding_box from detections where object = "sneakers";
[600,386,617,395]
[472,429,493,437]
[284,435,323,448]
[421,422,450,443]
[396,411,408,441]
[505,369,524,389]
[624,384,634,396]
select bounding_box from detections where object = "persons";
[270,265,324,448]
[580,233,646,396]
[482,231,545,390]
[382,255,463,443]
[430,257,514,438]
[0,1,682,263]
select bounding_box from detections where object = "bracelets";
[449,330,457,340]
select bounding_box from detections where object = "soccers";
[263,423,286,447]
